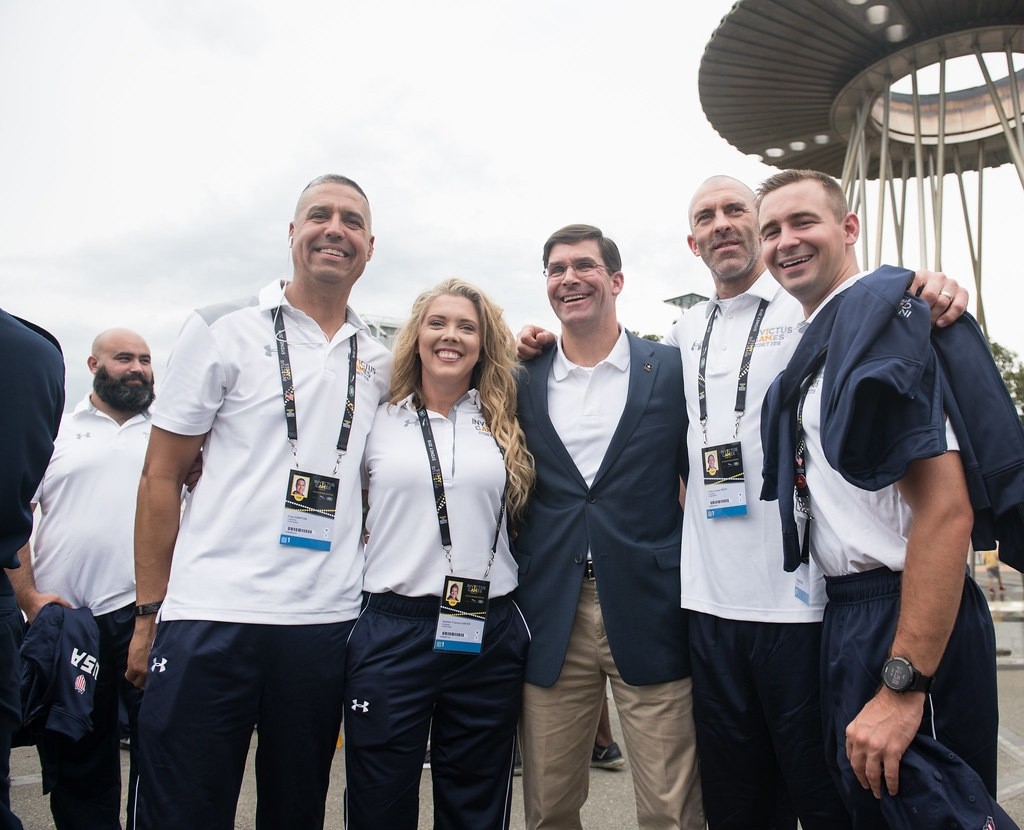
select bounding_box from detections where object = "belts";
[583,560,595,580]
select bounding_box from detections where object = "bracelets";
[134,600,163,616]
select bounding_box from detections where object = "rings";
[940,291,951,299]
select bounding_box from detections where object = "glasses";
[543,261,609,278]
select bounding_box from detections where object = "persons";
[0,174,1000,830]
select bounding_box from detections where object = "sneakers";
[514,751,524,776]
[588,742,624,768]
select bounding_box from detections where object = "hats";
[878,734,1021,830]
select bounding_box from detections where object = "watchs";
[881,656,932,694]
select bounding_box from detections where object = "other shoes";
[422,749,432,769]
